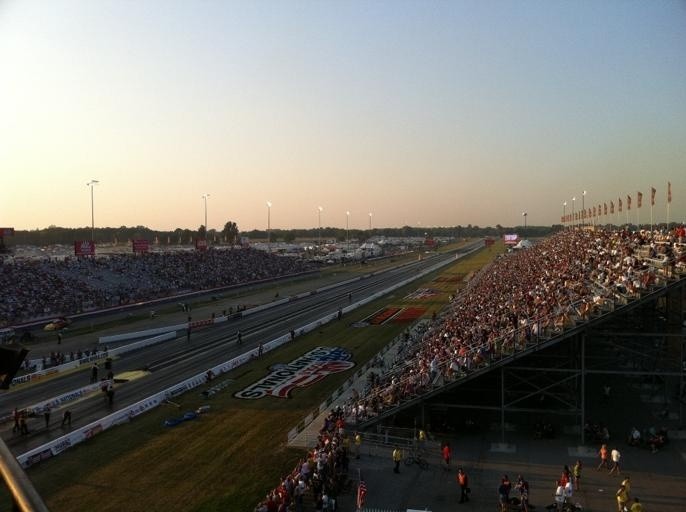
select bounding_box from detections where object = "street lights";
[368,212,373,237]
[0,342,30,391]
[572,196,575,231]
[582,190,587,229]
[318,207,322,254]
[265,202,272,255]
[563,201,567,231]
[523,213,528,240]
[346,211,350,250]
[86,179,100,241]
[202,194,211,231]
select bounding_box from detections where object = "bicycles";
[403,449,429,470]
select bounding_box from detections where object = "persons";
[497,473,530,512]
[290,326,295,344]
[442,440,453,471]
[314,221,685,464]
[235,328,244,345]
[628,496,643,511]
[0,245,315,338]
[622,475,632,512]
[573,458,583,491]
[250,428,369,511]
[416,453,429,471]
[608,444,625,476]
[0,338,117,439]
[182,323,192,346]
[456,466,471,504]
[393,444,403,474]
[205,369,216,384]
[551,464,574,511]
[257,341,264,360]
[615,484,628,511]
[595,443,611,471]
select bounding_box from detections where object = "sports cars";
[42,317,72,332]
